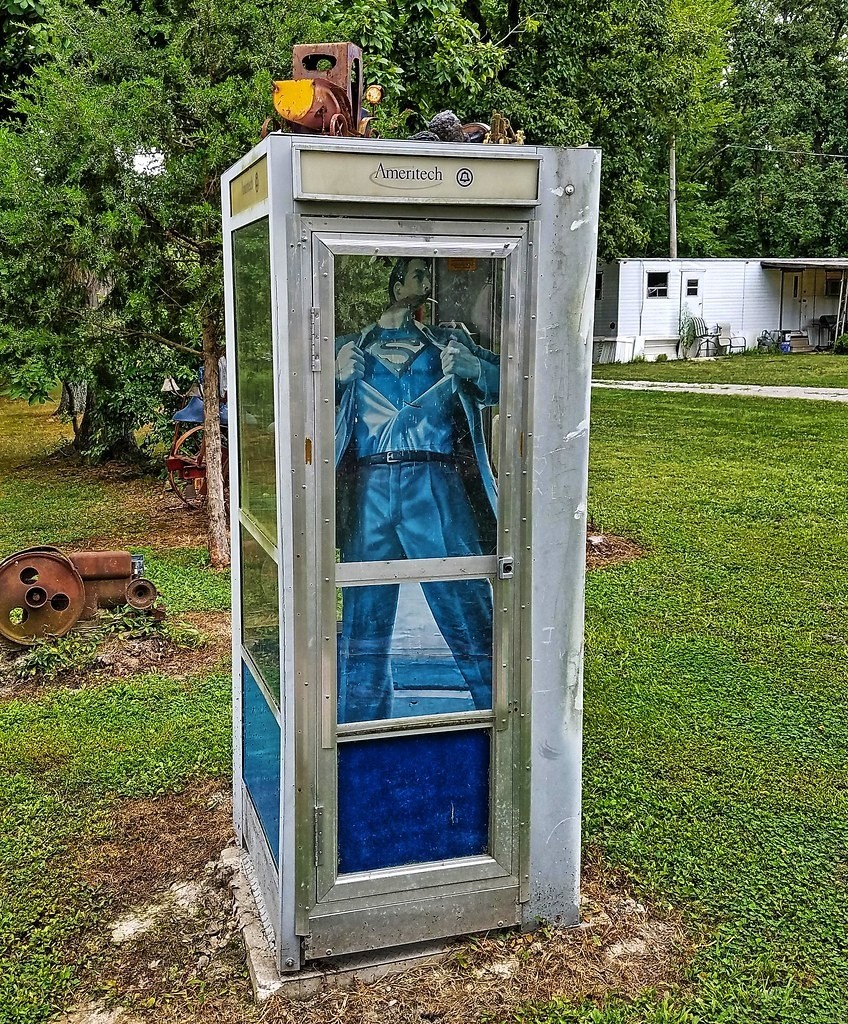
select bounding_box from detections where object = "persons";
[335,257,499,721]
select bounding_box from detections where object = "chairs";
[715,323,747,355]
[688,317,721,357]
